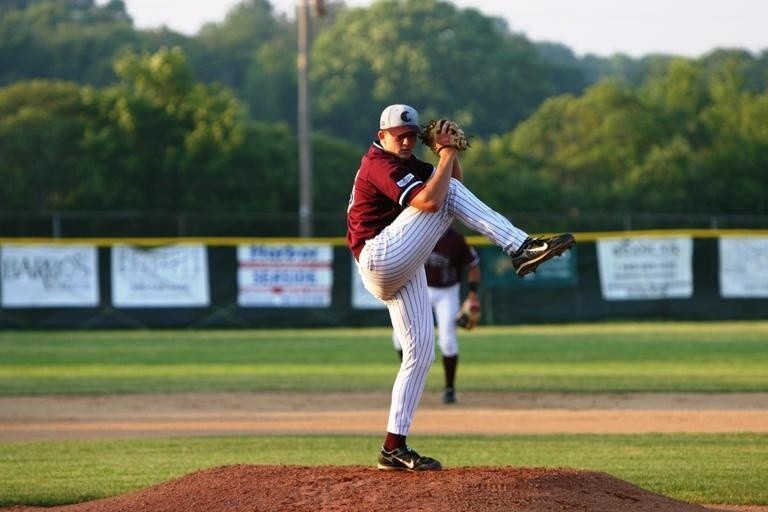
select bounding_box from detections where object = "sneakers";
[442,386,456,404]
[512,231,576,280]
[377,444,443,471]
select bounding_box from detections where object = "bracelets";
[466,280,481,295]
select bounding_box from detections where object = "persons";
[342,102,582,474]
[392,222,481,402]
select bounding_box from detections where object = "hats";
[379,103,422,137]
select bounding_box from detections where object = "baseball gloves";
[419,118,471,156]
[455,296,481,329]
